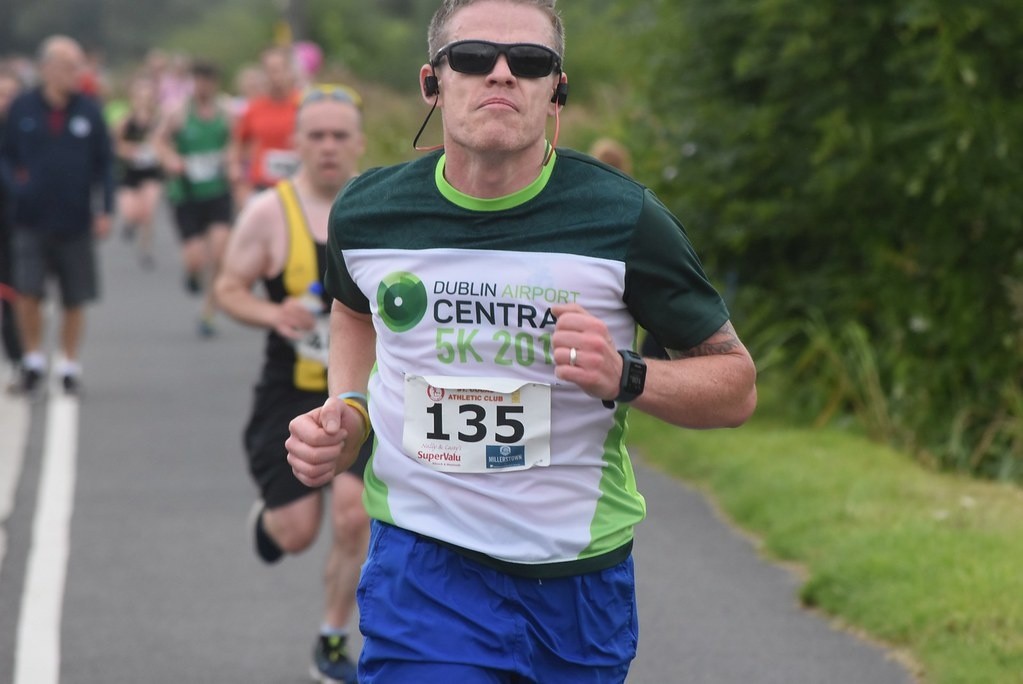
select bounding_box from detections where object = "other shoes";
[63,375,82,390]
[18,370,42,389]
[310,639,356,684]
[252,508,283,562]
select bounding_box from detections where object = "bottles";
[301,283,324,315]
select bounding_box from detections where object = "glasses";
[433,40,561,78]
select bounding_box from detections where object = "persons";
[283,0,758,684]
[153,35,324,336]
[0,33,192,397]
[211,83,377,684]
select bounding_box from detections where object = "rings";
[570,347,577,366]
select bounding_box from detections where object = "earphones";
[423,75,440,97]
[550,83,568,106]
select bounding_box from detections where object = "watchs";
[616,348,647,404]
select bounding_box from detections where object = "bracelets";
[336,392,367,403]
[344,398,370,441]
